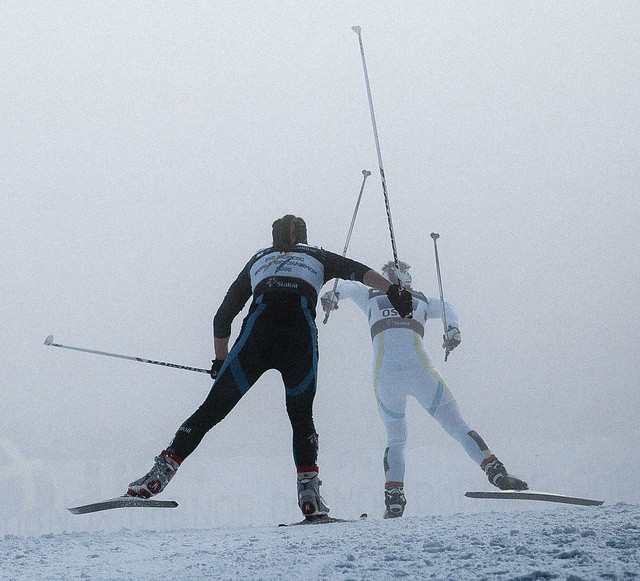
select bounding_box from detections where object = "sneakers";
[480,454,529,491]
[128,450,183,498]
[297,465,330,516]
[383,481,406,518]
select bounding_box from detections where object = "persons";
[129,215,413,515]
[321,260,529,518]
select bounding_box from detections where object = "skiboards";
[466,492,604,506]
[66,500,368,526]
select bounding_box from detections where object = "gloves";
[442,325,461,351]
[321,290,339,311]
[386,284,413,318]
[211,360,224,380]
